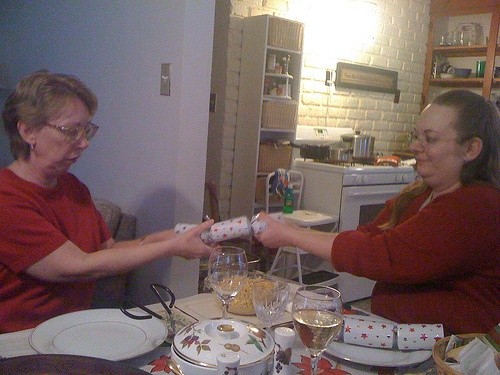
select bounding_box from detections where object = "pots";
[323,142,354,162]
[340,131,376,159]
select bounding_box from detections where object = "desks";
[0,294,433,375]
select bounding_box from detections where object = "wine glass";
[292,285,343,375]
[208,246,248,320]
[252,280,290,335]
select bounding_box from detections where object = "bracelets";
[139,235,146,245]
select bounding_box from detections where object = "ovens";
[292,159,415,303]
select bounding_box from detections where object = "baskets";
[433,334,500,375]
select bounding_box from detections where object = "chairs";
[265,170,313,284]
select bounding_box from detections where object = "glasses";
[42,121,99,141]
[411,132,466,147]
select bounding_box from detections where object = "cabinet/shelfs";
[238,14,304,273]
[419,0,500,113]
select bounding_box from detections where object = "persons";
[254,88,500,341]
[0,70,223,336]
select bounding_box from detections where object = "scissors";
[118,283,184,331]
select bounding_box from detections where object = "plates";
[324,315,432,367]
[29,308,168,361]
[439,73,455,78]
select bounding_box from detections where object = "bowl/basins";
[171,320,276,375]
[205,270,278,316]
[0,354,154,375]
[455,68,471,78]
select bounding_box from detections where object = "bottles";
[274,327,295,375]
[282,184,295,214]
[267,52,292,97]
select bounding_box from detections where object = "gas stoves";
[292,124,414,170]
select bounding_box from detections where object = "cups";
[476,61,485,77]
